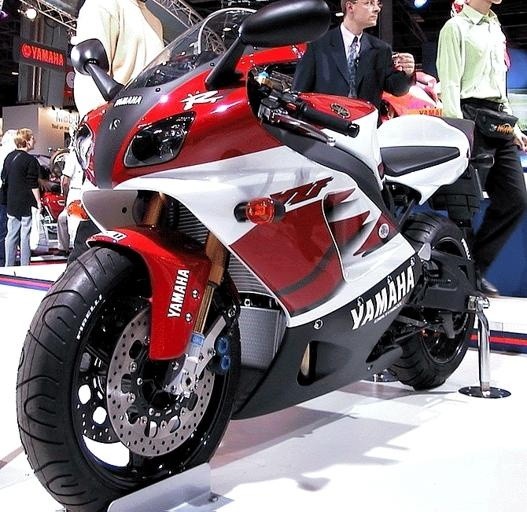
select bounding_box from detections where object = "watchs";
[15,0,494,512]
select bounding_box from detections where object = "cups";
[352,2,383,9]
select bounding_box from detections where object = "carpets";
[346,36,358,100]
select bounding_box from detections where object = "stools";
[462,99,505,111]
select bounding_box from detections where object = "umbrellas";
[55,249,70,256]
[480,278,499,297]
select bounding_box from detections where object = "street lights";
[461,105,519,140]
[0,181,9,204]
[27,205,50,252]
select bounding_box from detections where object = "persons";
[0,127,42,268]
[67,0,166,267]
[435,2,527,300]
[54,129,87,256]
[289,1,417,123]
